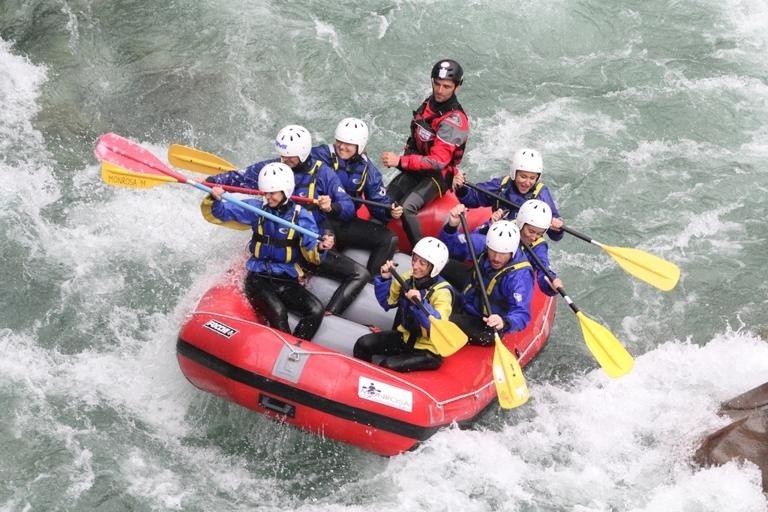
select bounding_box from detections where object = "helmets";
[483,218,523,257]
[507,146,545,183]
[515,196,554,238]
[271,123,314,166]
[255,159,297,202]
[428,57,466,87]
[333,115,371,156]
[411,234,452,279]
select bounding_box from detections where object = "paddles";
[100,157,322,209]
[166,143,399,213]
[455,205,530,410]
[95,133,330,244]
[383,261,468,358]
[456,172,680,291]
[492,208,634,378]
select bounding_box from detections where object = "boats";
[175,195,574,469]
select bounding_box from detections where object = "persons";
[379,58,469,244]
[209,162,336,342]
[452,147,563,241]
[442,203,535,347]
[351,234,453,372]
[473,198,562,297]
[203,124,370,316]
[308,115,402,286]
